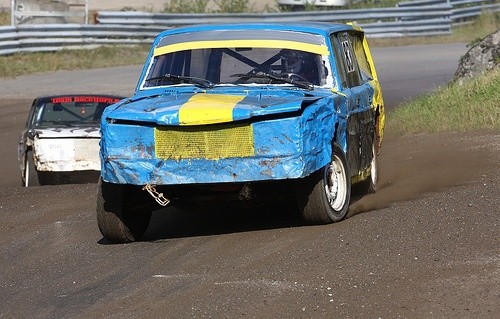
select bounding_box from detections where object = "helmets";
[279,48,304,73]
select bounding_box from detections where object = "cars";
[96,21,384,243]
[18,94,128,189]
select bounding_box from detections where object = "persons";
[271,49,320,86]
